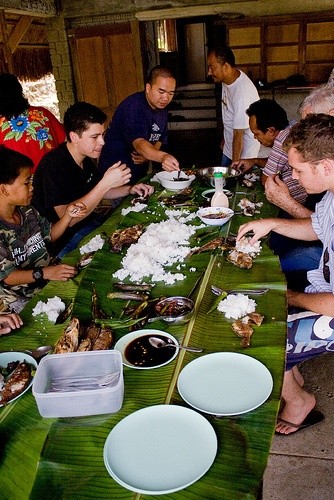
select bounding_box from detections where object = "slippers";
[274,410,325,437]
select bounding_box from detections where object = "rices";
[112,201,204,287]
[245,173,260,181]
[79,234,105,255]
[237,197,264,210]
[31,296,66,324]
[217,293,257,319]
[150,174,160,182]
[236,236,262,258]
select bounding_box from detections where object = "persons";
[98,66,180,210]
[0,71,67,186]
[236,112,334,435]
[207,43,274,167]
[0,313,23,336]
[31,101,154,262]
[0,144,88,315]
[280,69,334,289]
[230,98,327,258]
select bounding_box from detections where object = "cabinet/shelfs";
[224,9,334,91]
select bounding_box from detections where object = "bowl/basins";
[196,166,241,188]
[156,170,195,191]
[196,206,234,225]
[155,296,195,325]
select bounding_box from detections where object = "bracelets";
[129,186,133,195]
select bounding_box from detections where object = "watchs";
[32,266,44,282]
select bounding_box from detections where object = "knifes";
[197,228,220,243]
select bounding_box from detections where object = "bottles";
[211,172,229,208]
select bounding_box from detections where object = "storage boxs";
[32,349,124,418]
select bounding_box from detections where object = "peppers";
[207,291,228,314]
[90,282,161,332]
[196,230,219,241]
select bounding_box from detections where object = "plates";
[176,352,273,416]
[201,189,234,200]
[102,405,218,495]
[114,330,179,370]
[0,352,37,407]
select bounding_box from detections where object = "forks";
[49,371,119,392]
[211,285,269,297]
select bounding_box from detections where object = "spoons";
[149,337,203,353]
[6,345,54,358]
